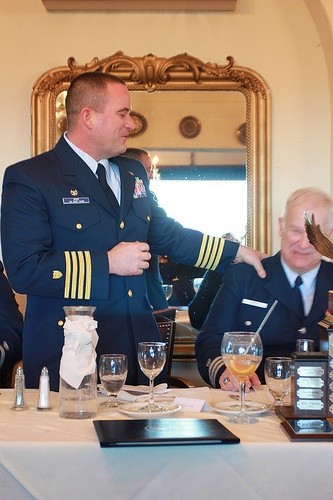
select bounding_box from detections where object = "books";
[93,419,239,449]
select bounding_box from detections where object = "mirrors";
[29,52,272,361]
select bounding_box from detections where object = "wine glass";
[98,354,127,408]
[221,331,263,424]
[264,356,292,411]
[138,341,167,411]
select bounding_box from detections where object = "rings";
[222,377,230,385]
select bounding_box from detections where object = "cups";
[297,339,314,352]
[193,278,205,292]
[162,284,172,300]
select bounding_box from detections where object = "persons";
[0,260,24,388]
[193,186,333,395]
[122,148,241,328]
[0,73,266,389]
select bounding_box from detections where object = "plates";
[123,111,148,139]
[180,115,201,138]
[118,402,181,419]
[235,121,246,145]
[207,399,274,416]
[56,116,68,135]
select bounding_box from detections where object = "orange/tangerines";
[229,355,256,375]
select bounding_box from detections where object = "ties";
[96,163,120,210]
[293,275,305,317]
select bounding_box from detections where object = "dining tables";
[0,388,333,500]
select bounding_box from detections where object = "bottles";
[58,305,97,418]
[37,367,51,413]
[11,365,28,412]
